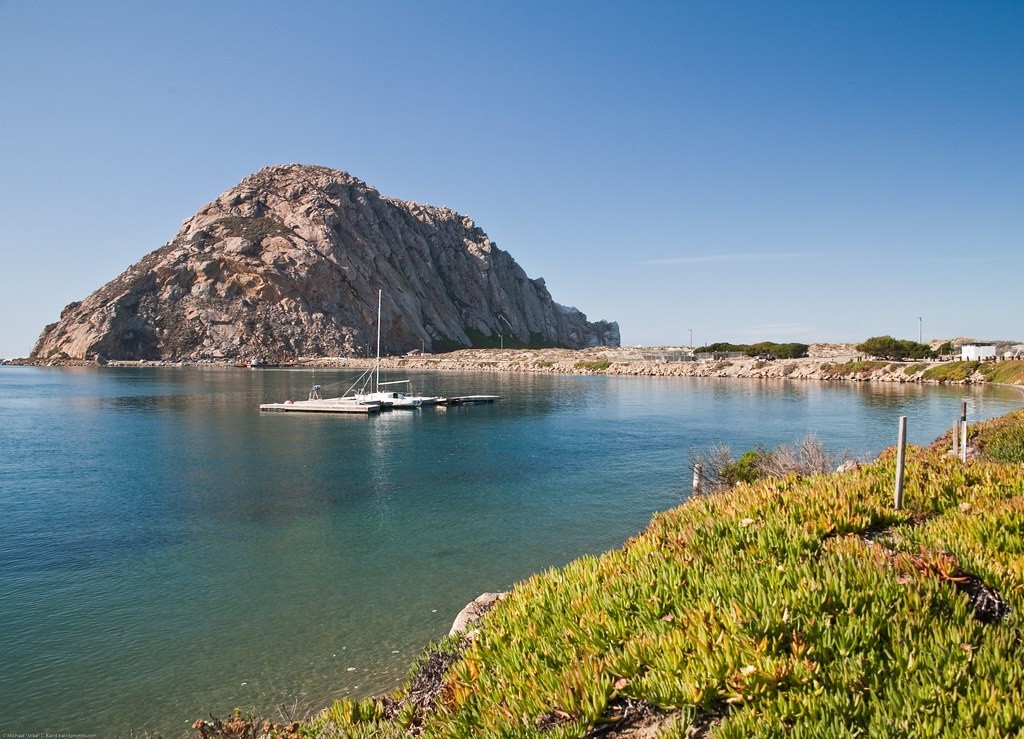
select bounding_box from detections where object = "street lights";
[688,328,692,362]
[498,334,504,359]
[916,316,922,345]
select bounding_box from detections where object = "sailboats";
[353,288,423,408]
